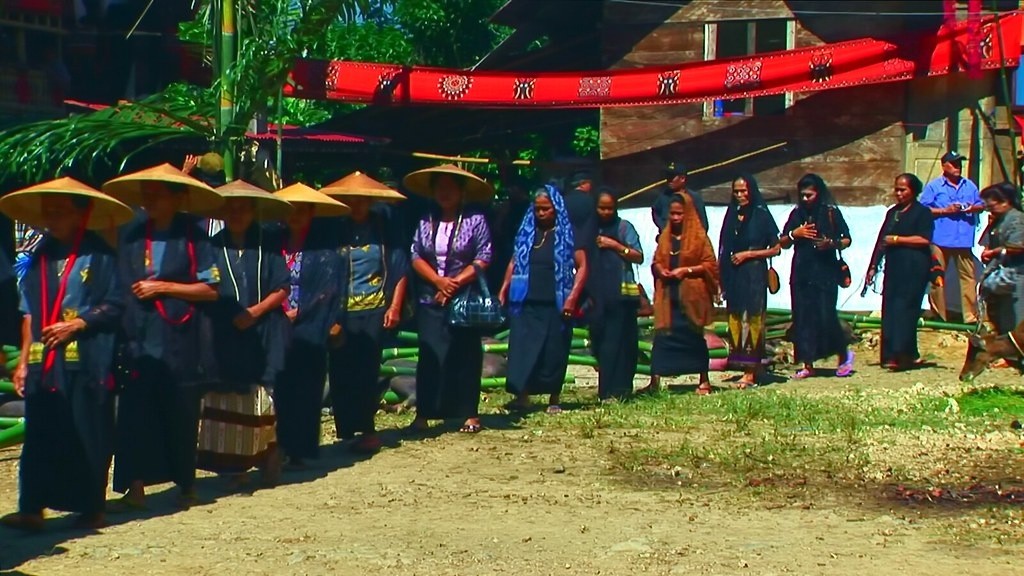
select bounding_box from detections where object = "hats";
[664,162,687,178]
[0,163,494,231]
[941,151,965,163]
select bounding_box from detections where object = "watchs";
[688,267,693,276]
[620,246,630,254]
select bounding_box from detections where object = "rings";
[564,312,568,315]
[568,313,572,317]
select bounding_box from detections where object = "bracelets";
[894,235,898,246]
[1001,245,1006,257]
[788,231,795,242]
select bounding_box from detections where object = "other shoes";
[335,437,381,452]
[178,493,198,510]
[1,513,45,528]
[883,361,910,370]
[105,498,151,513]
[911,359,925,367]
[69,515,108,530]
[218,476,289,494]
[546,403,563,414]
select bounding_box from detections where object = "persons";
[636,192,717,396]
[653,161,709,242]
[865,174,935,369]
[496,181,587,414]
[0,156,496,531]
[714,173,781,389]
[564,169,601,329]
[779,173,856,379]
[980,183,1024,368]
[578,187,645,403]
[919,151,988,326]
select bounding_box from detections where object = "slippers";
[794,367,817,380]
[694,386,711,396]
[836,351,855,376]
[730,376,759,389]
[459,422,482,434]
[398,425,429,439]
[501,402,528,415]
[634,384,660,395]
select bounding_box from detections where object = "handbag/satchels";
[447,265,507,332]
[976,247,1017,303]
[835,242,851,288]
[767,255,779,293]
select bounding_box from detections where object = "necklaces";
[47,258,68,280]
[670,251,680,256]
[671,233,687,241]
[532,230,548,248]
